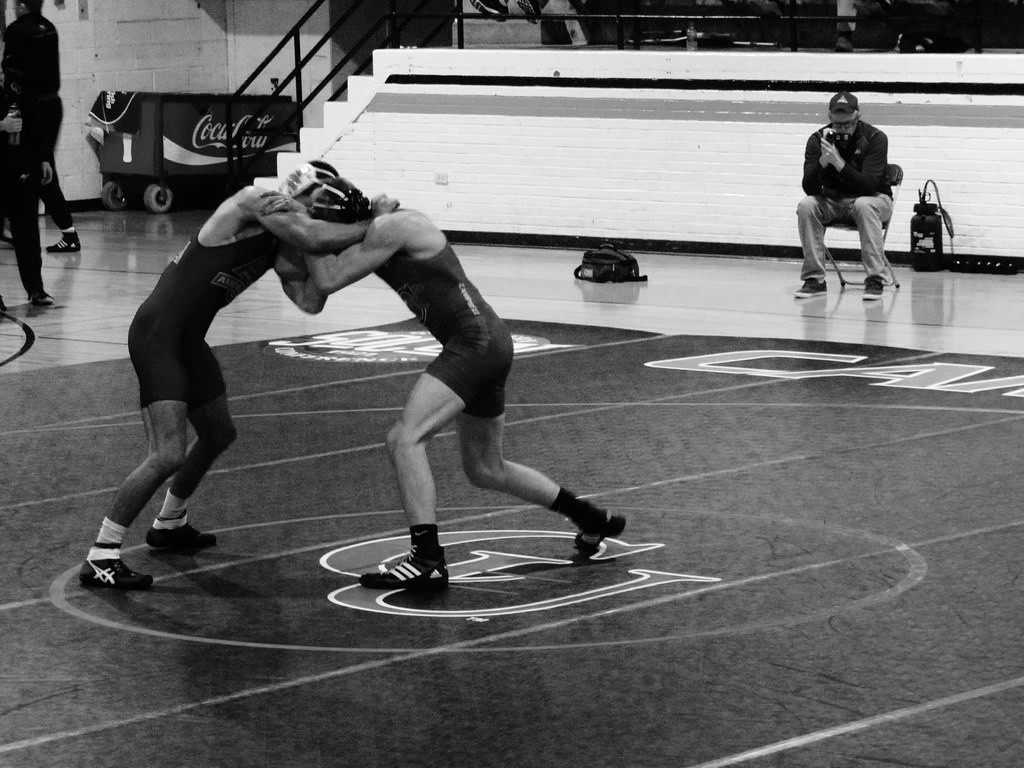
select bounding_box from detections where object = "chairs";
[796,164,904,289]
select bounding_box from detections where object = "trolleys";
[96,90,295,215]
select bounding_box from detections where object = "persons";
[796,92,893,300]
[0,0,81,253]
[0,86,54,317]
[470,0,549,24]
[78,161,400,587]
[835,0,860,51]
[260,178,627,589]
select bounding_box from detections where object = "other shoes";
[748,0,782,16]
[470,0,510,23]
[835,37,853,52]
[27,290,54,305]
[517,0,541,24]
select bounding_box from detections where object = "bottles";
[8,106,20,145]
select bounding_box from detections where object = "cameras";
[827,129,852,144]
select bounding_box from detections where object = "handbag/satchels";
[573,244,648,283]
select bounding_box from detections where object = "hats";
[829,90,859,112]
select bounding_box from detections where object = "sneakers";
[574,508,625,553]
[862,278,883,299]
[359,545,449,588]
[46,230,81,252]
[795,277,827,297]
[79,558,154,589]
[145,523,217,550]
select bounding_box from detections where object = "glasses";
[830,117,858,128]
